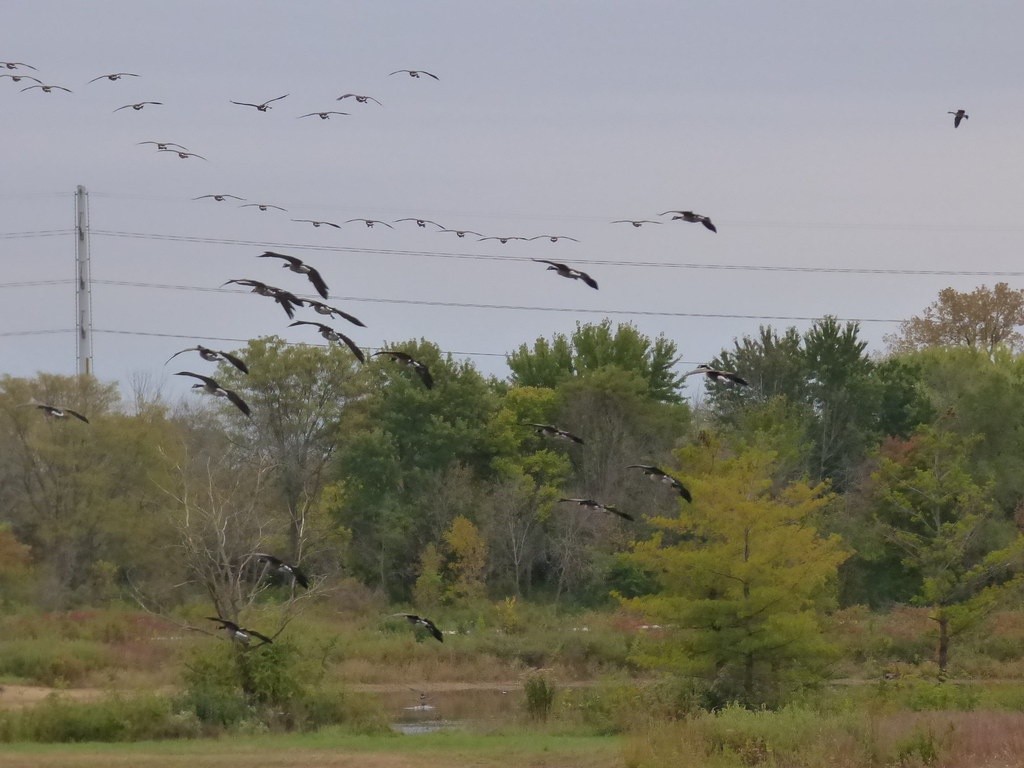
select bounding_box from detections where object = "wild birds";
[0,60,164,114]
[205,616,273,645]
[532,259,599,290]
[556,498,635,521]
[164,344,252,420]
[15,403,91,425]
[610,211,718,234]
[229,69,440,121]
[372,352,434,390]
[948,110,969,128]
[516,422,586,446]
[624,464,692,505]
[218,252,369,365]
[685,364,750,388]
[384,612,444,643]
[238,552,312,590]
[193,194,583,245]
[138,141,208,161]
